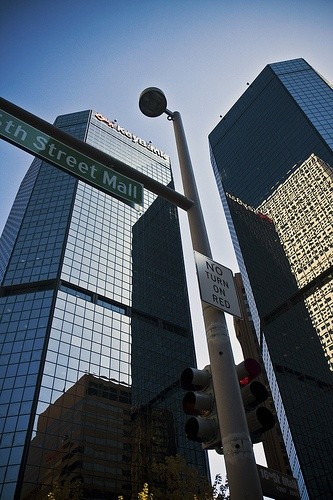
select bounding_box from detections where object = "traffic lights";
[179,364,224,450]
[233,357,276,441]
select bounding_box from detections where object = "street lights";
[138,86,267,499]
